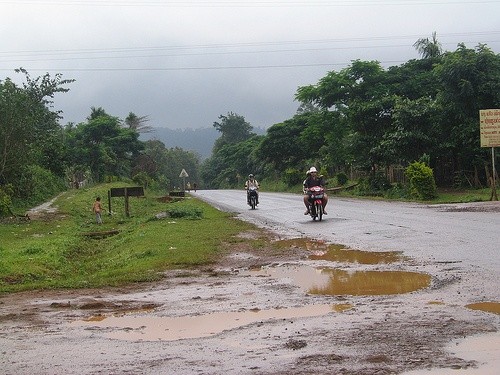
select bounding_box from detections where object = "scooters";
[302,182,330,222]
[244,184,261,210]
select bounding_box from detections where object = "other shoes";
[304,210,310,215]
[323,210,328,215]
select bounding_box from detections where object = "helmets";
[306,167,317,175]
[249,174,253,176]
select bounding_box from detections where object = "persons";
[92,197,102,223]
[186,181,190,191]
[194,182,197,192]
[245,174,259,205]
[303,167,328,215]
